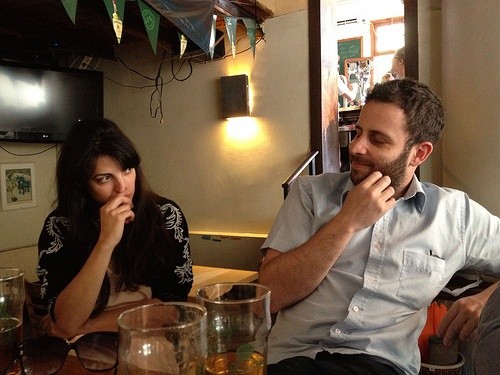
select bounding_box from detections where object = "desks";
[188,263,259,305]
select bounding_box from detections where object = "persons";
[38,118,194,340]
[338,76,359,101]
[382,46,405,83]
[256,78,500,375]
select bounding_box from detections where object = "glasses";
[18,331,119,375]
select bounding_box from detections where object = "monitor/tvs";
[0,60,103,143]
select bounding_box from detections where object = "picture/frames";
[0,162,36,211]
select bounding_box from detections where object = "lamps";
[219,74,250,120]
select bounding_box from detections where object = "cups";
[196,281,271,375]
[115,302,208,375]
[0,267,26,375]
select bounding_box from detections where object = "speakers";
[220,74,250,118]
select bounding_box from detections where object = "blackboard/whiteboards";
[338,36,364,108]
